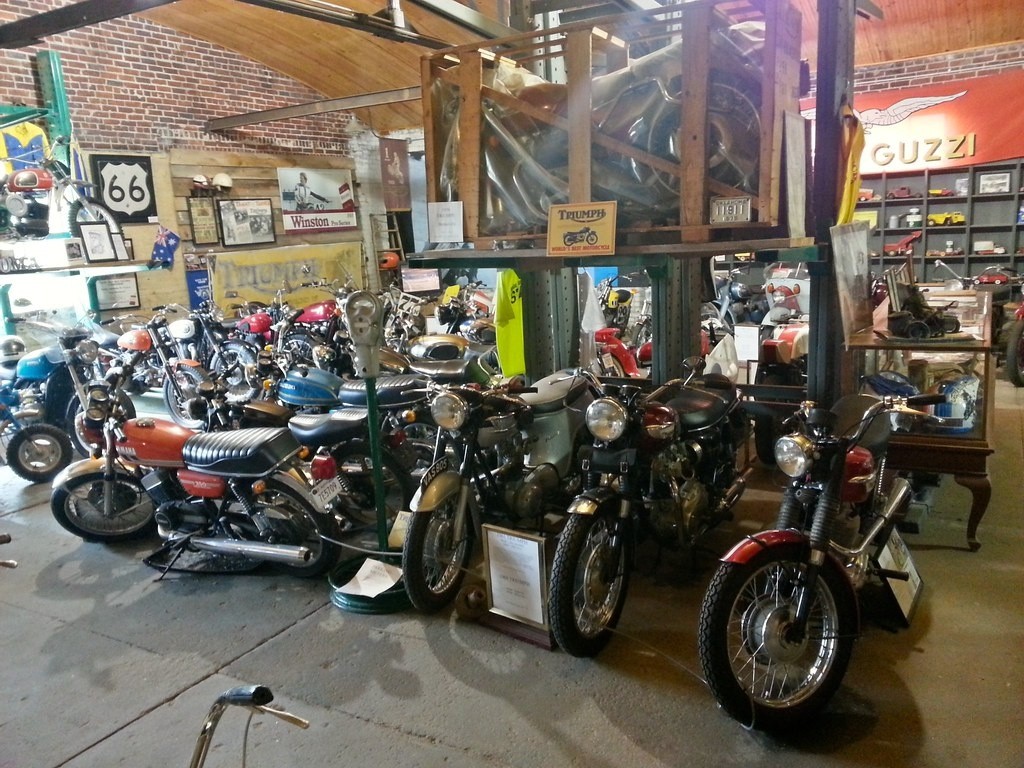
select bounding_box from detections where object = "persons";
[292,172,332,211]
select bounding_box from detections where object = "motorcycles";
[697,372,950,733]
[0,267,811,619]
[931,259,1023,388]
[545,357,755,660]
[0,133,128,260]
[437,18,810,230]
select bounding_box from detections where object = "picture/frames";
[217,198,277,248]
[187,196,219,246]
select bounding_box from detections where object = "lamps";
[343,112,366,138]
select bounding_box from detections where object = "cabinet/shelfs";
[841,287,994,552]
[855,156,1024,302]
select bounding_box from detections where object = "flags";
[147,225,180,273]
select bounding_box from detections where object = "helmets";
[192,175,211,190]
[212,173,232,191]
